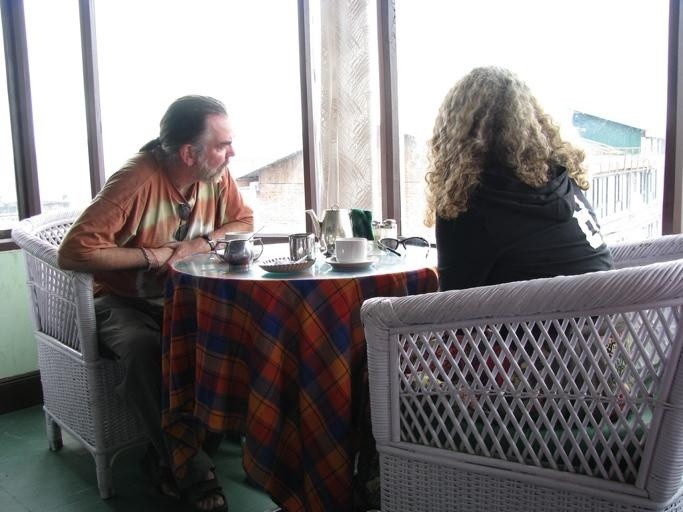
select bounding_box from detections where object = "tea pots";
[303,203,354,256]
[207,237,263,264]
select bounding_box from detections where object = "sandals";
[136,442,230,512]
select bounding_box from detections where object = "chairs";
[10,203,149,502]
[357,233,682,511]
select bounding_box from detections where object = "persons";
[353,66,636,512]
[56,95,255,512]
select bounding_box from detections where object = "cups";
[287,233,316,261]
[225,231,253,246]
[333,237,372,260]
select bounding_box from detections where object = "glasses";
[175,201,192,242]
[376,236,431,260]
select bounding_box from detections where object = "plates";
[325,256,377,270]
[258,256,311,274]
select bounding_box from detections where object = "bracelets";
[141,246,159,273]
[202,234,215,250]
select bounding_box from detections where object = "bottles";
[371,218,397,249]
